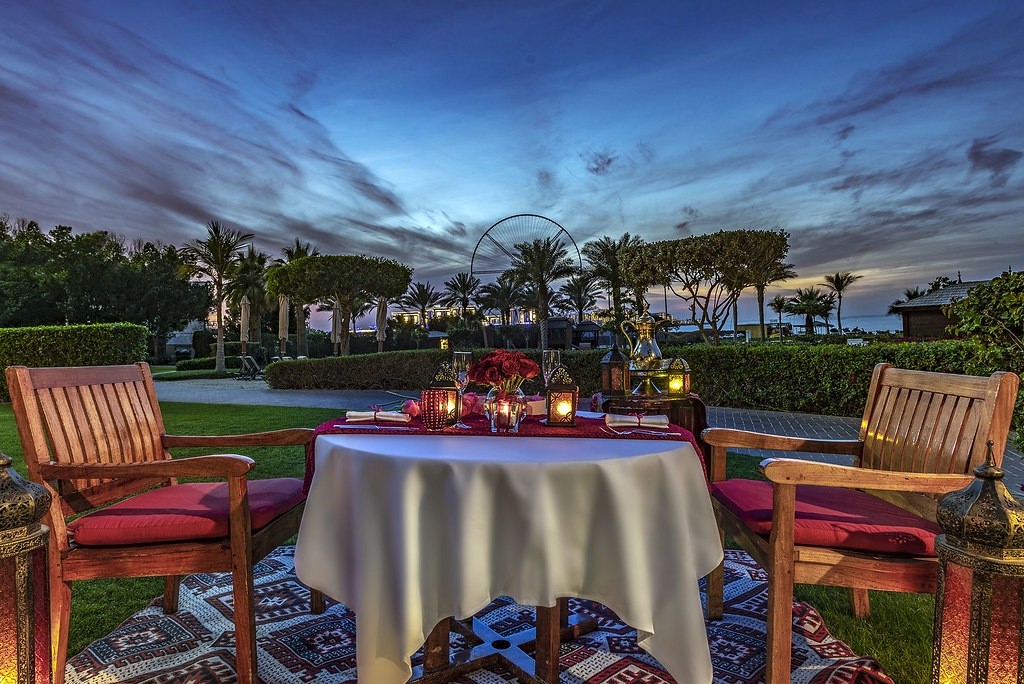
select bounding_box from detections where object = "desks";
[589,391,710,477]
[294,411,724,684]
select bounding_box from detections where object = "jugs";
[620,304,667,370]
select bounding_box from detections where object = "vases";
[490,401,519,433]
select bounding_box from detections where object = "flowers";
[466,349,541,396]
[400,393,487,418]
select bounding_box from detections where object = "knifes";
[333,425,419,430]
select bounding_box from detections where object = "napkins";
[605,415,669,428]
[345,410,412,423]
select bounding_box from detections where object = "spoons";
[607,425,681,435]
[599,425,667,437]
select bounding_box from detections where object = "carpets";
[64,543,896,684]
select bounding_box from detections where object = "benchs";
[701,362,1019,684]
[6,361,327,684]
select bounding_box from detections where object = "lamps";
[429,361,462,425]
[933,438,1024,684]
[667,357,691,398]
[546,364,579,427]
[0,452,53,684]
[598,336,632,399]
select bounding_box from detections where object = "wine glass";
[450,352,472,430]
[539,350,560,423]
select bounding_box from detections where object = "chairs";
[237,355,308,381]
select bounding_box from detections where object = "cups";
[490,401,520,432]
[421,389,448,432]
